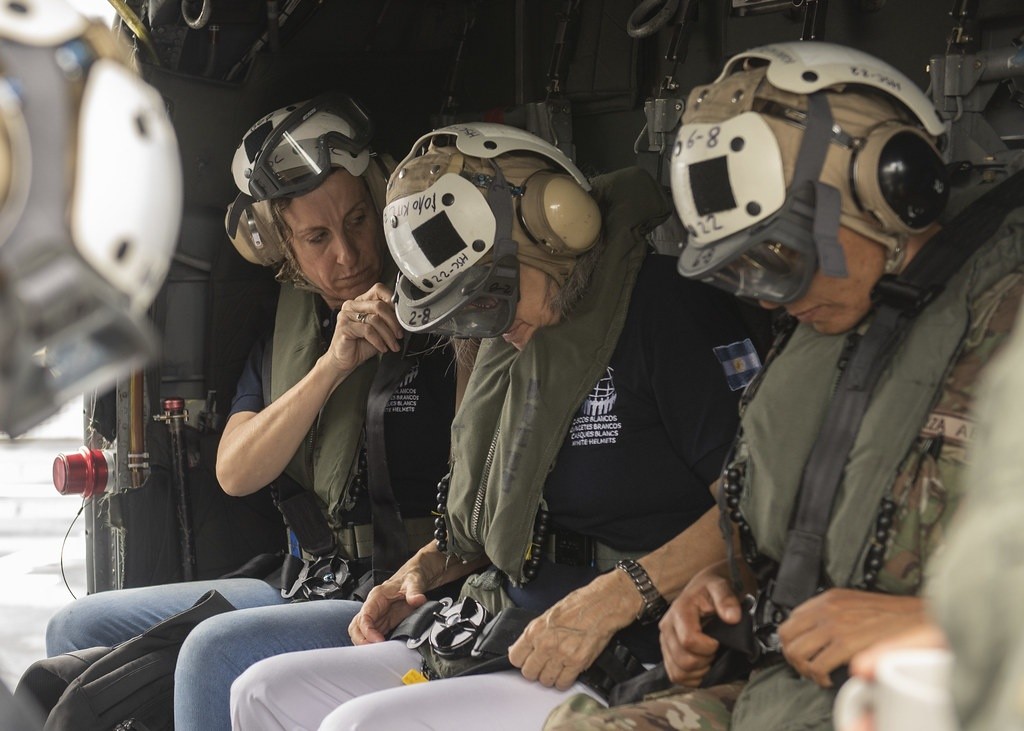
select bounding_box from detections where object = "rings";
[356,312,372,323]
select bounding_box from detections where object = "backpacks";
[12,587,238,731]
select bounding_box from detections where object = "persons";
[45,114,480,731]
[540,40,1024,731]
[228,123,768,731]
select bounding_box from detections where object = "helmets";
[669,39,947,276]
[382,121,591,292]
[230,97,381,261]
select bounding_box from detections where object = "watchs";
[615,558,668,626]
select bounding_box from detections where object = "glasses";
[227,93,376,239]
[677,93,847,308]
[391,158,520,340]
